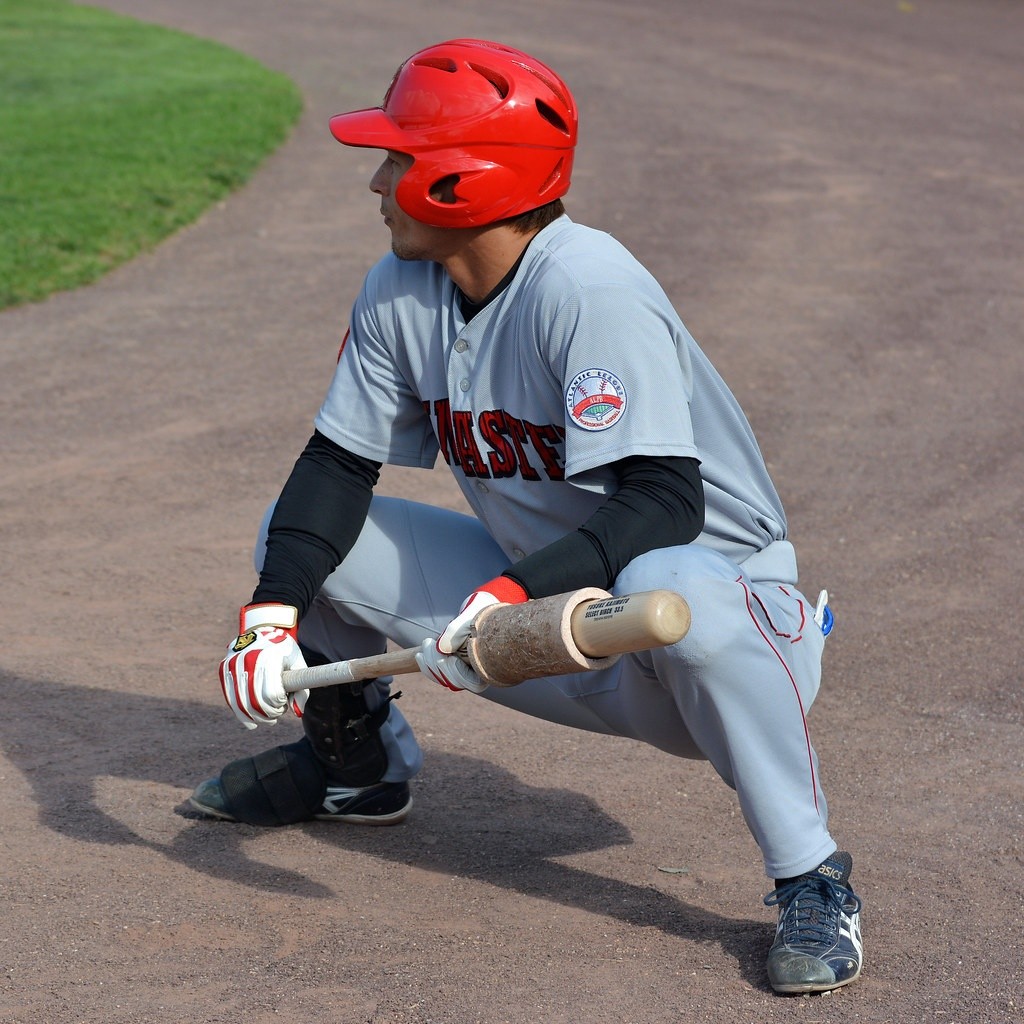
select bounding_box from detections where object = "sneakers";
[189,757,413,826]
[767,852,863,993]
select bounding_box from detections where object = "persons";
[182,38,866,994]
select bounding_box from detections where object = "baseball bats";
[278,591,692,694]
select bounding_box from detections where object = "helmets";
[330,38,578,227]
